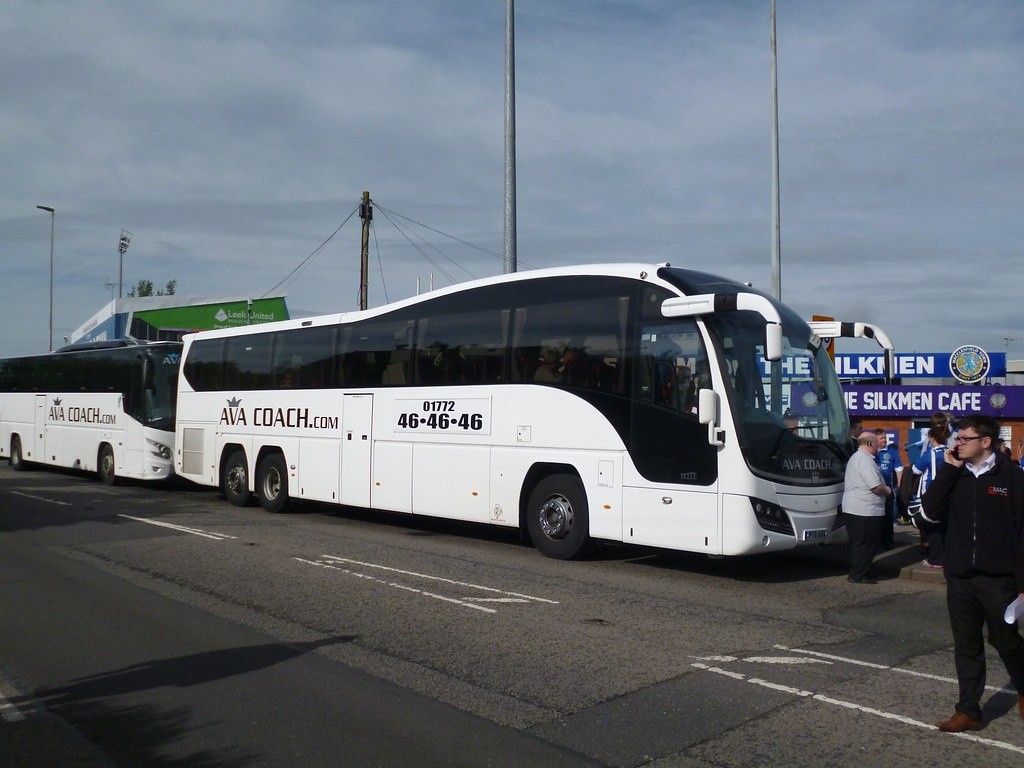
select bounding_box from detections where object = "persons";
[911,412,1024,732]
[534,327,587,387]
[841,428,903,584]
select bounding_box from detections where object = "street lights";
[37,205,55,354]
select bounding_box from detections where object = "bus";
[174,262,895,560]
[0,340,183,485]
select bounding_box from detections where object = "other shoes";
[847,576,877,584]
[1018,695,1024,718]
[921,560,943,568]
[934,711,983,732]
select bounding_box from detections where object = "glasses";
[954,435,986,445]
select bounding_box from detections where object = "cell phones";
[951,446,963,461]
